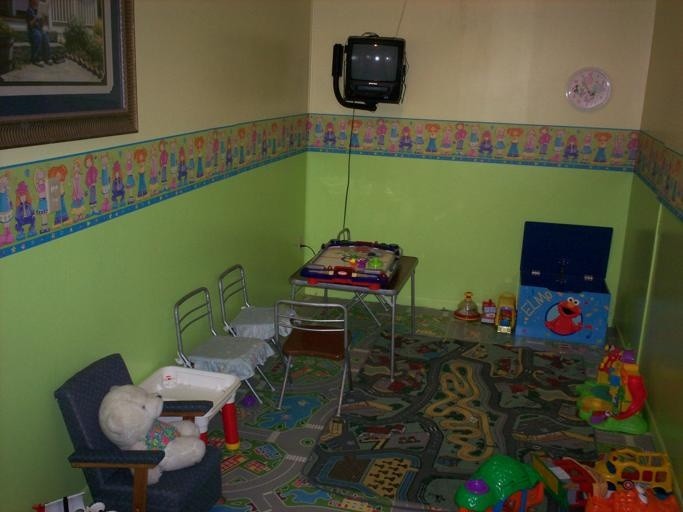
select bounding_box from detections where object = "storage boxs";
[515,221,613,347]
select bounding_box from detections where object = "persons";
[26,0,54,66]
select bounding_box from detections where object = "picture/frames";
[0,0,138,150]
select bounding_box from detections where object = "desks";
[289,243,418,383]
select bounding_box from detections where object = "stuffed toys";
[97,382,207,486]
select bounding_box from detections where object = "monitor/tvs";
[343,36,407,104]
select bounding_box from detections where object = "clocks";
[565,68,612,112]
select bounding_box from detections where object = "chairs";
[274,299,352,417]
[53,354,222,511]
[336,228,389,327]
[218,264,297,369]
[174,287,275,404]
